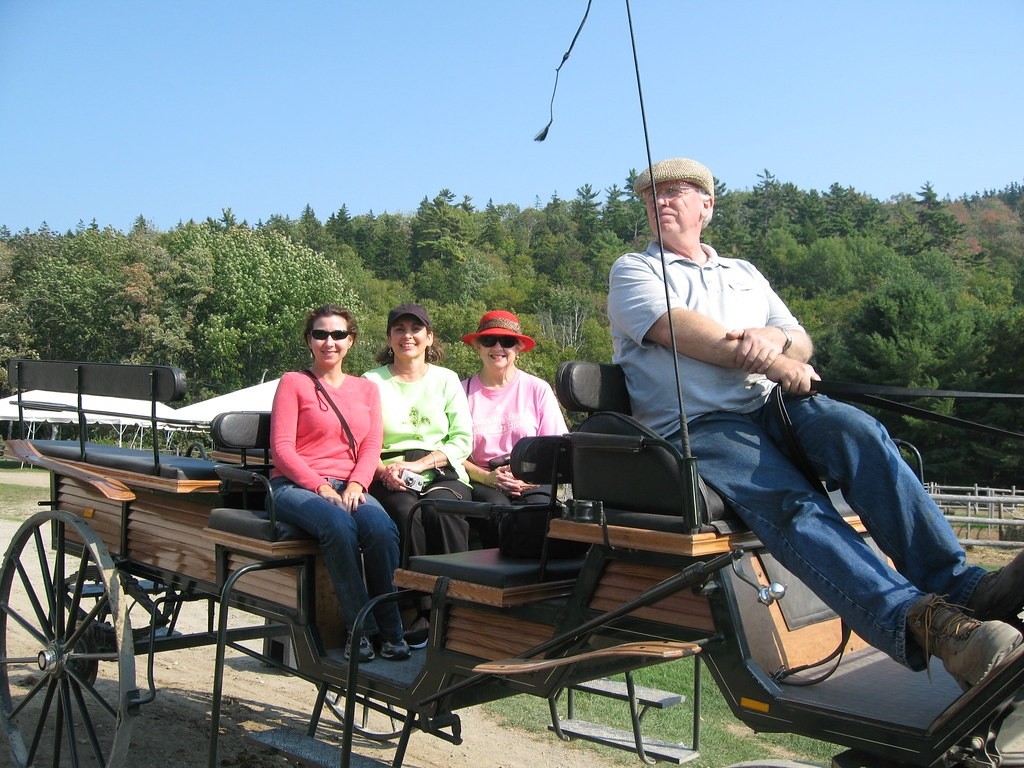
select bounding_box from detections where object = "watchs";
[777,325,793,353]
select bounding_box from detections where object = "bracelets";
[431,453,446,476]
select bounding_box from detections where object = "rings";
[500,489,504,493]
[348,497,353,501]
[392,488,394,491]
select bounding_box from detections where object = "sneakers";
[345,624,375,661]
[378,637,411,659]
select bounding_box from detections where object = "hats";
[387,304,431,336]
[462,310,535,352]
[633,158,714,201]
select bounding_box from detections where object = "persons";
[266,306,569,659]
[609,157,1024,696]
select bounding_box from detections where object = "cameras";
[400,471,424,492]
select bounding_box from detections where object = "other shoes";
[404,608,430,649]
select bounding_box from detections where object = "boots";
[964,549,1024,621]
[907,593,1024,693]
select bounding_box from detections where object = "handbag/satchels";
[496,491,566,557]
[488,452,511,471]
[381,449,459,488]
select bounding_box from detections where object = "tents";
[0,375,282,471]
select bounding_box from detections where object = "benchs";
[3,358,243,503]
[513,362,923,547]
[209,411,322,562]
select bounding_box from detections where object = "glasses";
[312,330,352,340]
[646,185,703,204]
[480,336,518,348]
